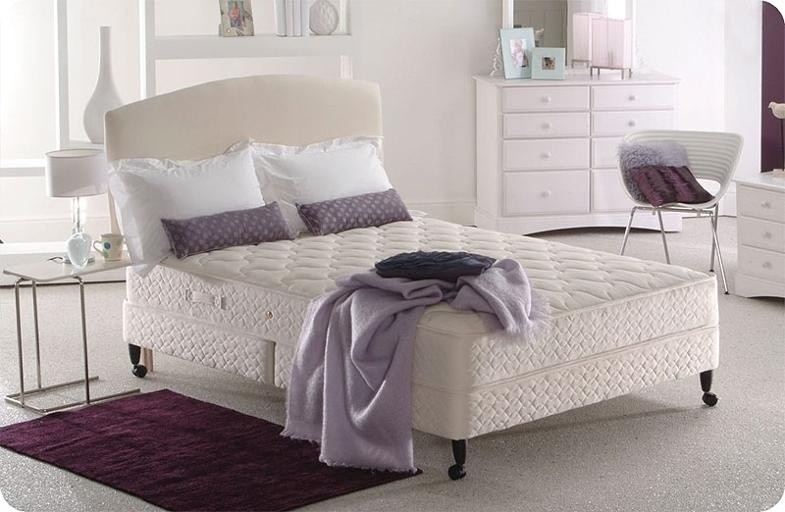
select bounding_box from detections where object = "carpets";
[0,390,424,511]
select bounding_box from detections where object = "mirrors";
[502,1,639,72]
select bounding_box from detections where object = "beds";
[101,73,720,481]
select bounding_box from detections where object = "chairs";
[617,129,744,295]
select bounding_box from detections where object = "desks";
[3,252,149,415]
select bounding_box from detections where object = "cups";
[92,231,126,262]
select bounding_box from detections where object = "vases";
[81,26,124,144]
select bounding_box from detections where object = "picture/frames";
[498,27,535,79]
[218,0,253,37]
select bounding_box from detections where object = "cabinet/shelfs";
[529,47,565,80]
[471,71,684,237]
[730,168,785,299]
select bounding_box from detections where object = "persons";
[228,2,241,27]
[511,39,530,68]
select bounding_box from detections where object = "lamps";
[44,147,106,266]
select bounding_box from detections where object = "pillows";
[626,163,716,206]
[294,186,413,238]
[161,201,291,259]
[616,140,690,204]
[105,136,267,278]
[251,132,425,238]
